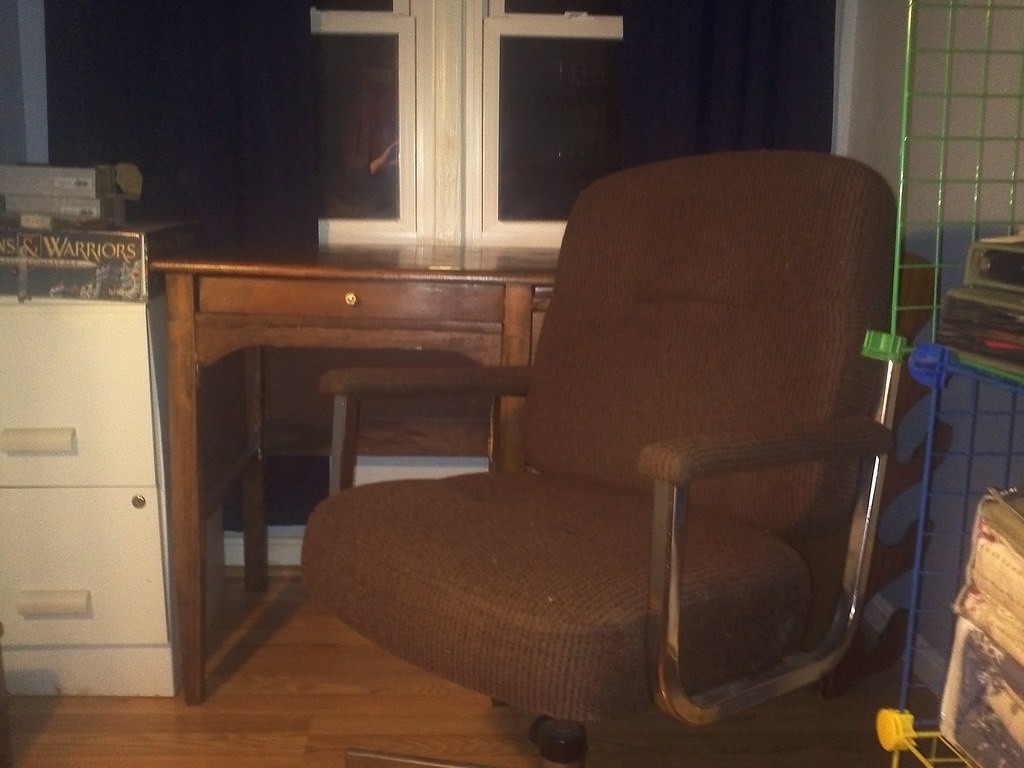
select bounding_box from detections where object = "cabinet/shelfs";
[1,282,208,697]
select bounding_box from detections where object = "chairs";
[300,149,902,766]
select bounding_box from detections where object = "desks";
[144,235,565,709]
